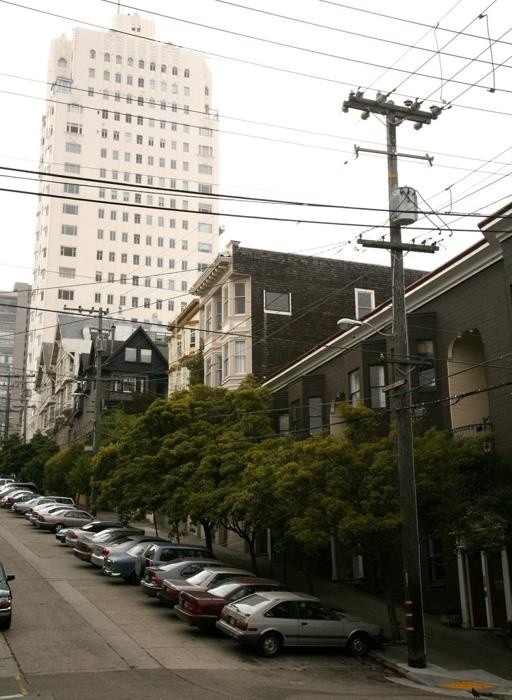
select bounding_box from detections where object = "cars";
[0,561,16,630]
[0,479,94,533]
[90,534,172,568]
[156,567,257,607]
[140,557,236,598]
[172,576,289,633]
[71,527,146,563]
[56,520,126,548]
[103,542,153,586]
[215,591,383,658]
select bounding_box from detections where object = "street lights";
[335,93,442,668]
[63,304,109,515]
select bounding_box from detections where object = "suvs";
[135,542,220,583]
